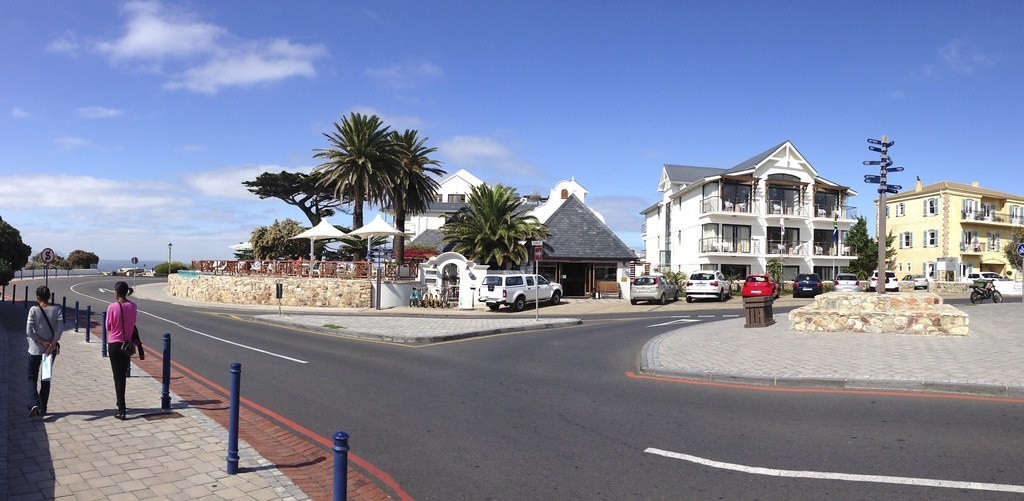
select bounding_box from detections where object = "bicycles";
[409,283,450,308]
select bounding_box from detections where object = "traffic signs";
[863,138,904,194]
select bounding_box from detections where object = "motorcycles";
[969,279,1003,304]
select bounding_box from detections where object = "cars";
[833,273,861,291]
[740,273,779,299]
[901,274,929,291]
[866,270,901,292]
[967,272,1004,281]
[630,274,680,305]
[793,273,822,298]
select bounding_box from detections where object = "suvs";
[685,269,732,302]
[478,274,563,313]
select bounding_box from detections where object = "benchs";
[596,282,622,299]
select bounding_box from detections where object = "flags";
[833,219,838,242]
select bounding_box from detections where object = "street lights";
[168,242,173,275]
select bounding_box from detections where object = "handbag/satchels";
[56,342,60,354]
[121,339,136,359]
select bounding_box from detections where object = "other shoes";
[40,409,47,416]
[115,410,125,420]
[28,404,40,417]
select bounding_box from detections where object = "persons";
[26,285,64,418]
[106,281,137,421]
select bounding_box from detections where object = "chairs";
[710,242,729,252]
[752,278,756,281]
[844,246,850,256]
[777,244,786,254]
[644,279,650,283]
[725,201,733,211]
[819,209,826,217]
[815,246,823,255]
[209,261,356,279]
[773,204,781,213]
[738,203,746,212]
[709,276,714,279]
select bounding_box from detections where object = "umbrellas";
[228,242,254,260]
[288,218,357,240]
[348,214,407,268]
